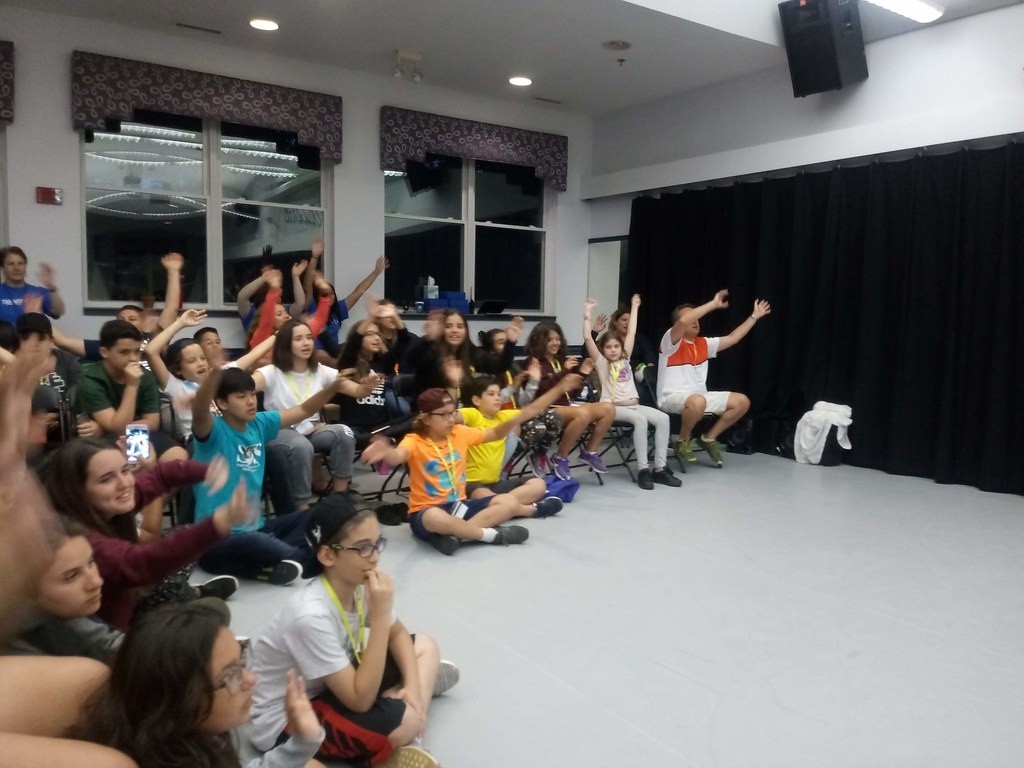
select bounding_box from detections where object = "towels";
[793,400,853,465]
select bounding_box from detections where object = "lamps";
[390,46,424,84]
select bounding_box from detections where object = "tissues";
[423,276,439,299]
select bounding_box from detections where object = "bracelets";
[311,256,317,259]
[750,314,755,319]
[270,332,277,336]
[49,287,56,292]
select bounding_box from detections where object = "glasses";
[333,537,387,558]
[431,410,459,419]
[198,638,253,695]
[364,330,384,340]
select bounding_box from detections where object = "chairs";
[71,331,741,530]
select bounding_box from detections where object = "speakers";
[404,165,433,198]
[778,0,869,99]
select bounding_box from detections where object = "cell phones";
[126,424,150,464]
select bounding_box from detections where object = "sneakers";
[550,452,571,480]
[675,438,697,462]
[695,434,723,465]
[500,460,513,480]
[526,453,548,479]
[577,448,609,474]
[530,496,563,518]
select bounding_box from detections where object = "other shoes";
[263,559,303,586]
[637,468,654,490]
[431,660,460,698]
[487,525,529,548]
[197,574,238,600]
[375,745,439,768]
[651,467,682,487]
[431,534,459,556]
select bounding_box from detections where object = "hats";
[418,388,454,414]
[301,490,373,579]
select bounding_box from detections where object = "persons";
[58,602,326,768]
[0,236,770,481]
[0,331,141,768]
[359,374,586,555]
[188,347,357,588]
[0,519,127,661]
[577,291,683,490]
[452,378,563,521]
[247,489,460,768]
[29,438,249,601]
